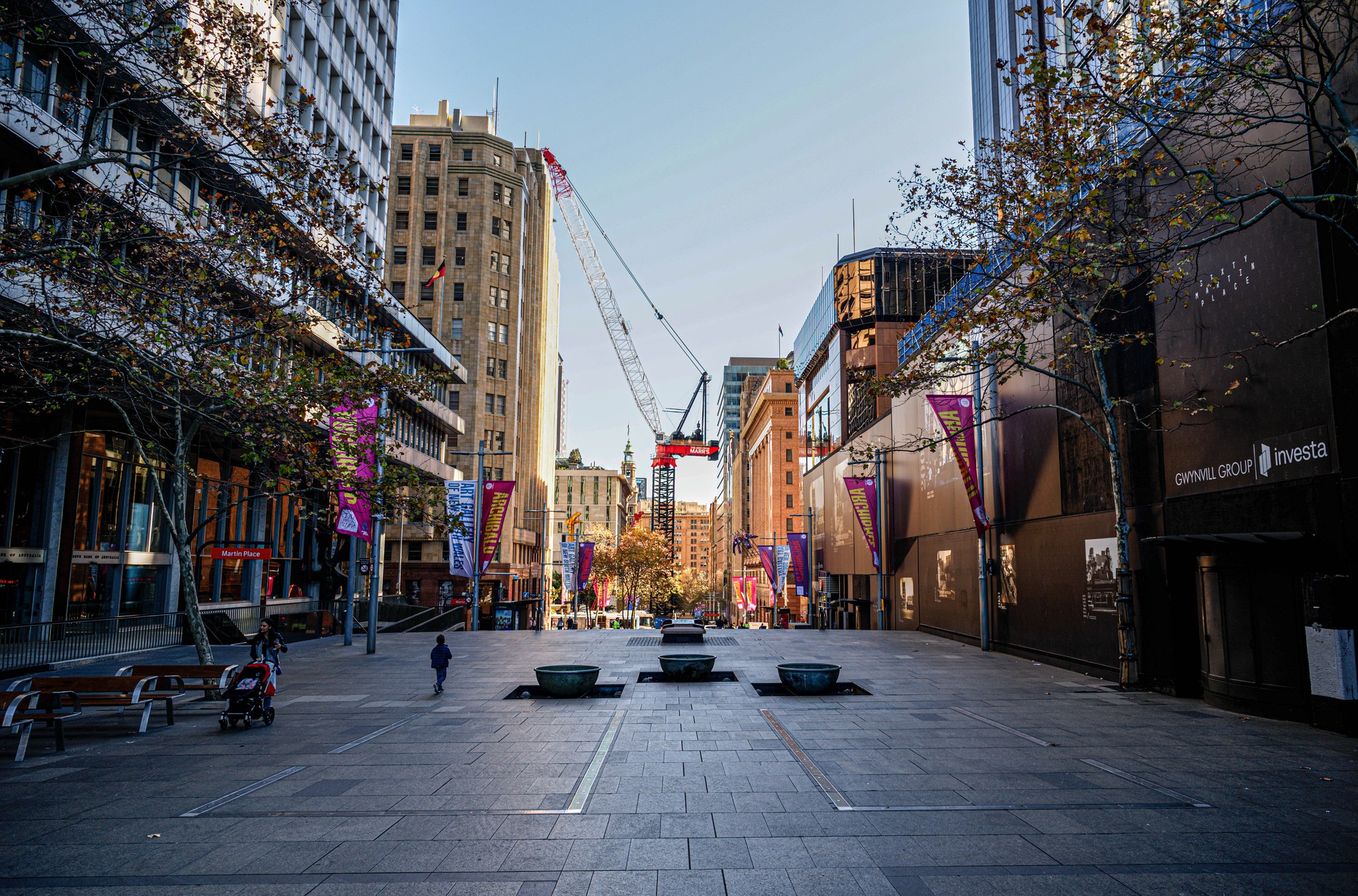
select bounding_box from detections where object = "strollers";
[218,658,276,730]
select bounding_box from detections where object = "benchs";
[661,619,706,643]
[0,664,240,762]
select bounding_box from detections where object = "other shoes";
[433,683,439,693]
[439,688,445,693]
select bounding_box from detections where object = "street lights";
[756,532,788,626]
[521,503,572,630]
[789,507,814,629]
[842,448,886,631]
[929,339,990,650]
[445,440,517,632]
[339,329,434,653]
[701,590,721,620]
[558,528,593,628]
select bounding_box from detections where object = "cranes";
[543,147,720,617]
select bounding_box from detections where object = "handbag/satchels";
[276,665,282,675]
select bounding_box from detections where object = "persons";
[801,619,805,623]
[660,622,663,631]
[409,592,420,606]
[496,615,511,629]
[556,617,566,630]
[761,623,766,629]
[716,616,750,629]
[778,620,784,629]
[683,611,693,616]
[613,617,620,629]
[430,634,453,693]
[530,617,536,628]
[566,616,573,629]
[250,618,288,720]
[795,619,800,623]
[589,625,592,629]
[445,589,452,603]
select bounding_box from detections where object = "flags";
[425,259,446,287]
